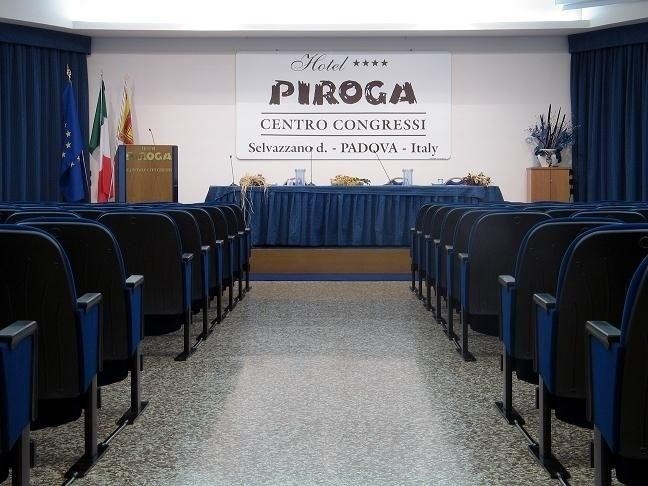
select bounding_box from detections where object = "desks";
[205,186,505,279]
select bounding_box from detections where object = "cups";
[438,178,443,183]
[402,167,413,186]
[294,168,306,187]
[551,159,559,167]
[286,179,293,186]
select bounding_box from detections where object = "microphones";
[310,147,313,183]
[374,150,391,181]
[149,128,155,145]
[229,155,234,183]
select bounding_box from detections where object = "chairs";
[0,199,180,214]
[213,206,246,303]
[423,206,459,324]
[197,206,238,312]
[509,199,646,219]
[225,204,251,292]
[17,218,150,426]
[415,205,442,316]
[444,208,506,341]
[528,223,648,479]
[495,218,624,425]
[161,210,213,360]
[0,320,38,483]
[586,255,648,486]
[184,207,213,341]
[187,208,228,324]
[456,211,552,363]
[409,205,432,292]
[99,211,198,361]
[433,207,471,331]
[0,224,110,486]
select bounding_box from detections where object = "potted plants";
[524,103,582,167]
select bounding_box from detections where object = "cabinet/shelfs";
[526,167,572,202]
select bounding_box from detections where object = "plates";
[283,184,295,187]
[431,182,445,186]
